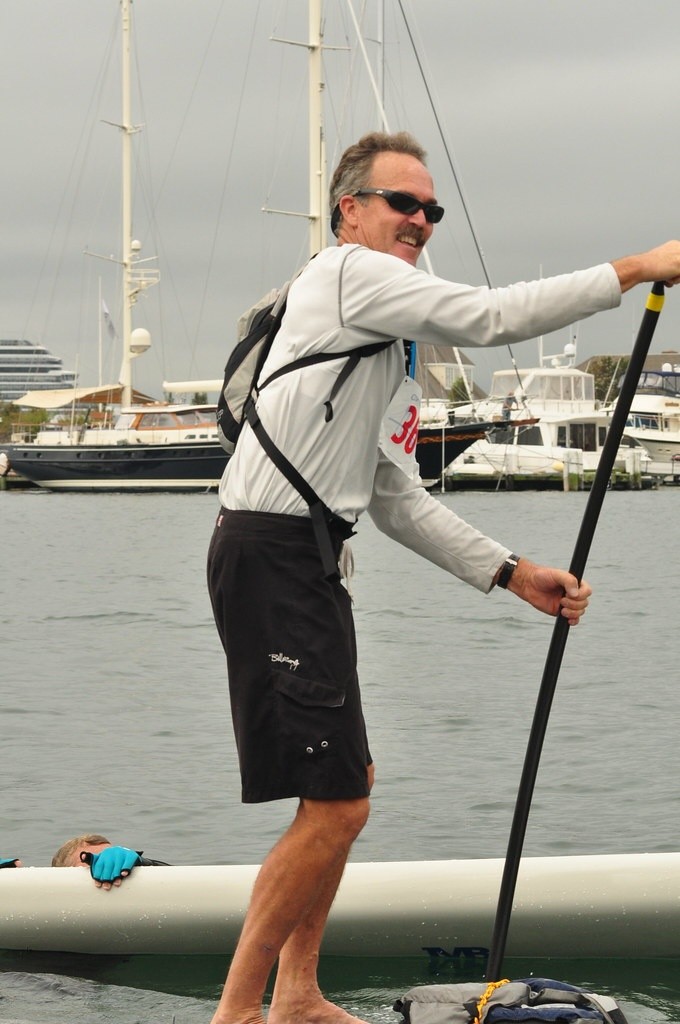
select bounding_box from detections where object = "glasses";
[351,186,446,223]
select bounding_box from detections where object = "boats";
[598,361,680,486]
[442,262,655,492]
[1,399,536,494]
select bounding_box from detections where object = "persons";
[0,834,176,890]
[206,128,680,1024]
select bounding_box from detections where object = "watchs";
[497,552,521,590]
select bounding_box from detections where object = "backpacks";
[216,281,290,457]
